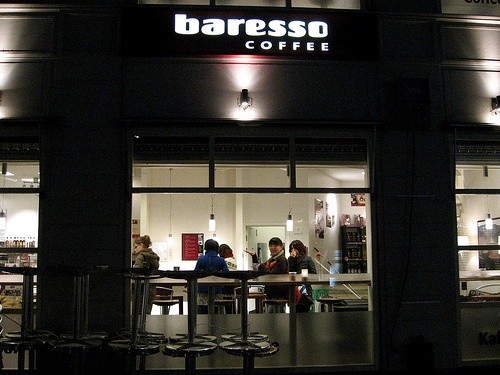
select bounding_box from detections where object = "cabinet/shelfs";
[338,223,369,275]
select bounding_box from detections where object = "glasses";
[134,242,145,249]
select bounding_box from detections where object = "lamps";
[208,198,216,231]
[286,208,293,231]
[0,208,7,230]
[485,214,494,229]
[239,88,248,108]
[490,96,500,113]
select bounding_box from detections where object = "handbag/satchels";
[295,286,302,304]
[300,283,313,305]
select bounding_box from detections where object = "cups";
[289,272,297,282]
[301,268,308,282]
[174,267,180,271]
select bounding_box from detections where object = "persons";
[193,239,230,314]
[288,240,317,313]
[132,235,160,315]
[244,236,288,312]
[219,244,237,270]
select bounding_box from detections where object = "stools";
[318,298,342,313]
[0,263,288,375]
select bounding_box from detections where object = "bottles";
[347,247,361,259]
[347,263,361,273]
[4,237,35,248]
[347,232,361,242]
[8,254,37,268]
